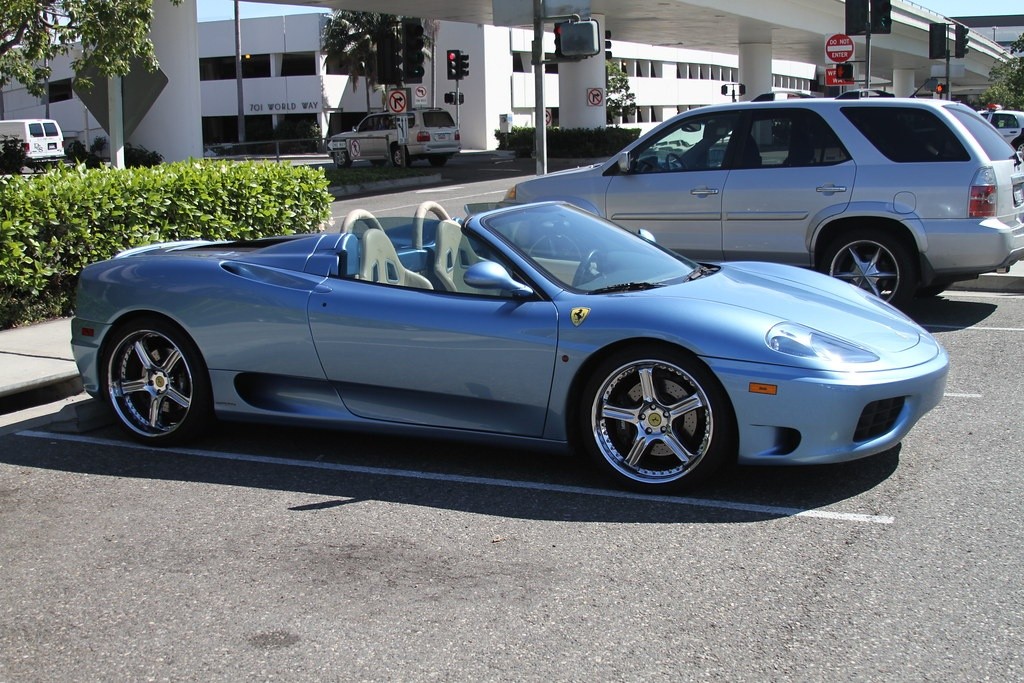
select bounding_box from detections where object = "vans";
[0,118,67,171]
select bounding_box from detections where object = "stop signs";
[825,34,854,61]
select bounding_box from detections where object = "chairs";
[992,116,1000,128]
[433,219,501,295]
[783,118,816,165]
[720,130,763,165]
[360,228,434,290]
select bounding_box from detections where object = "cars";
[976,103,1024,154]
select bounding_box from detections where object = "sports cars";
[69,196,953,496]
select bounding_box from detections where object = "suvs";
[496,88,1024,307]
[329,105,462,168]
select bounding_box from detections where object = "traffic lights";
[447,50,461,80]
[402,17,425,83]
[955,24,970,59]
[835,62,854,79]
[936,83,945,95]
[460,51,470,79]
[376,29,402,85]
[554,22,563,56]
[605,30,613,60]
[444,93,455,103]
